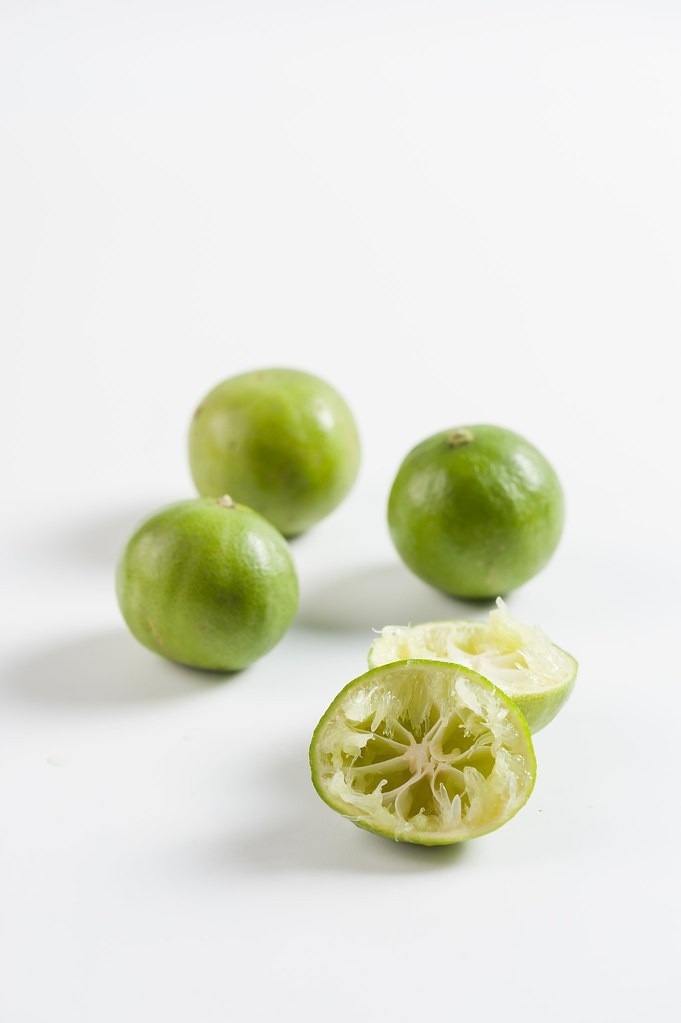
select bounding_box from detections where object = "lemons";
[116,369,579,845]
